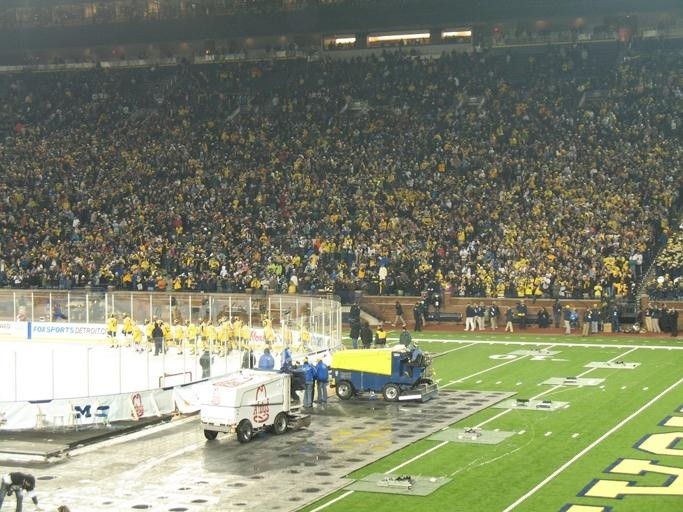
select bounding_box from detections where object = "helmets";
[22,474,36,492]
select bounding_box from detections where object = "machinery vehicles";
[192,368,315,444]
[326,343,442,403]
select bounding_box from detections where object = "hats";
[109,312,269,326]
[466,300,598,309]
[295,356,323,368]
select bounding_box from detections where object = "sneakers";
[109,343,273,358]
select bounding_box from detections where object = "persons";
[0,0,683,337]
[110,336,413,406]
[0,469,39,512]
